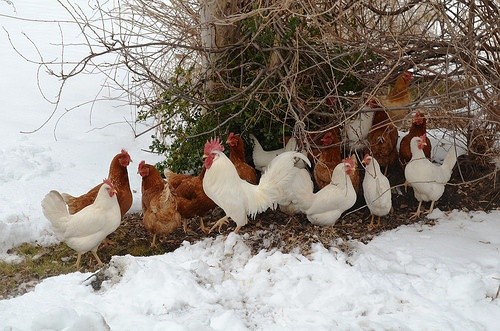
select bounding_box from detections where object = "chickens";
[41,69,460,270]
